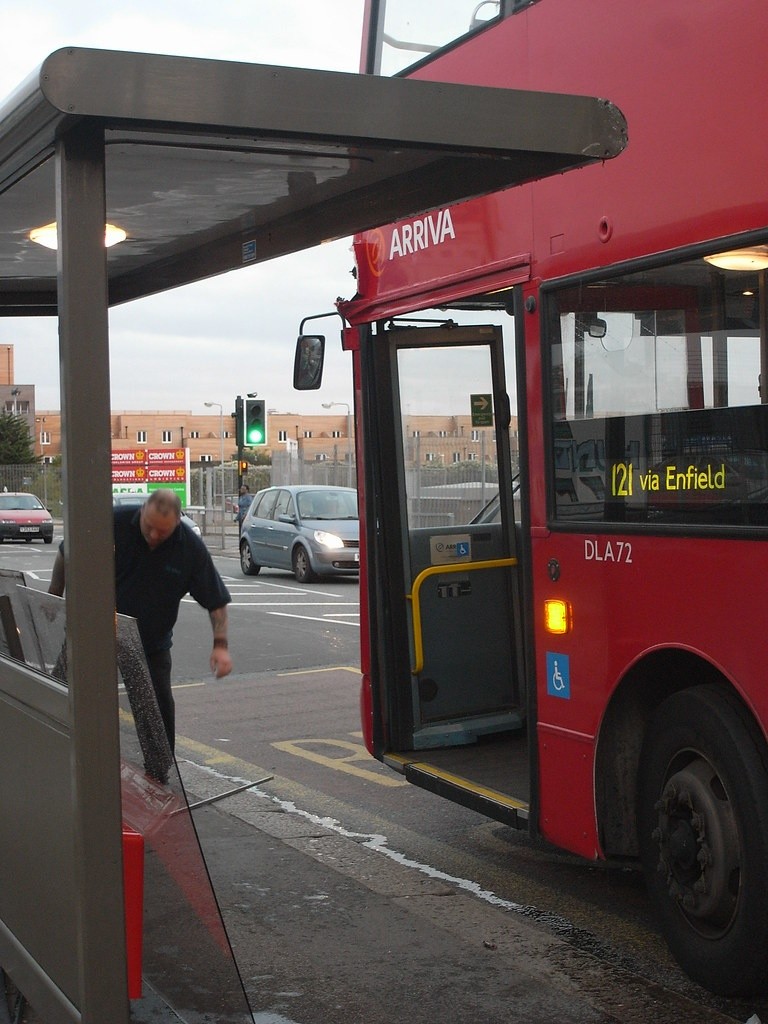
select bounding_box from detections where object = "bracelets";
[213,638,228,650]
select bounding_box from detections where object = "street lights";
[321,401,353,487]
[204,402,226,551]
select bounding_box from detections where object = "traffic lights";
[244,399,268,446]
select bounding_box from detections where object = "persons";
[233,484,255,524]
[39,489,233,797]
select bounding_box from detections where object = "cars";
[238,485,360,584]
[224,495,239,511]
[113,492,202,541]
[0,491,54,545]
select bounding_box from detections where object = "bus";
[291,0,768,1007]
